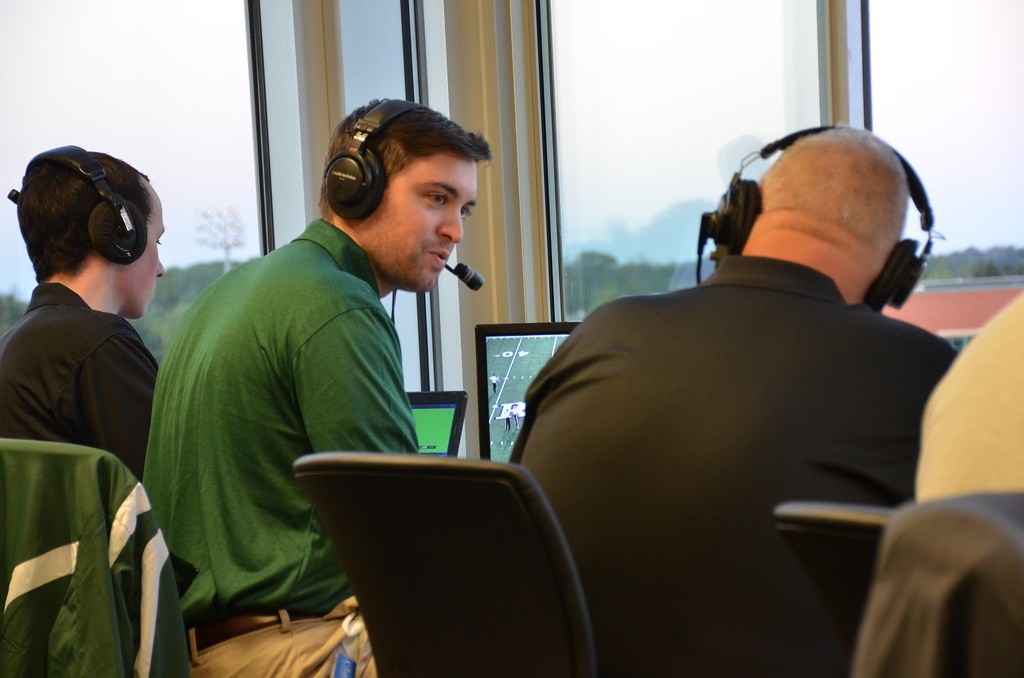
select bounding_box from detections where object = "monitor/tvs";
[476,321,585,464]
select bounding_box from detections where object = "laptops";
[403,390,470,458]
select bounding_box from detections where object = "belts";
[186,609,328,657]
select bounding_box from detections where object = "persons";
[0,145,167,484]
[142,99,492,678]
[490,373,521,431]
[506,127,961,678]
[915,288,1024,678]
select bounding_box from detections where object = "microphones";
[444,263,484,291]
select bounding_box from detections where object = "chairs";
[0,436,191,678]
[770,494,897,678]
[292,450,597,678]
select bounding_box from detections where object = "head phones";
[7,145,148,264]
[321,100,429,220]
[706,126,937,316]
